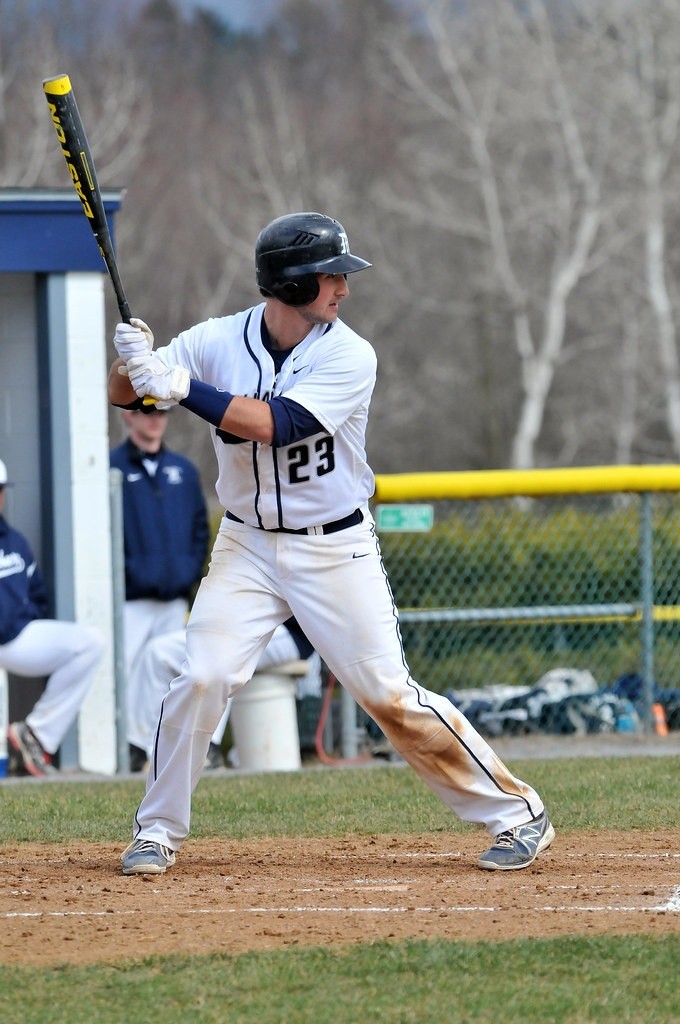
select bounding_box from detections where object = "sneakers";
[476,809,556,870]
[119,838,176,874]
[9,722,61,778]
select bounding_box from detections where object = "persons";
[108,396,211,773]
[125,616,317,774]
[108,211,556,874]
[0,460,102,775]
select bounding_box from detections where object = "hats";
[0,461,13,488]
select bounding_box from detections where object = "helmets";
[255,212,373,307]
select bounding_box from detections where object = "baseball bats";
[40,72,162,417]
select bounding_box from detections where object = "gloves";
[114,318,155,362]
[119,354,189,410]
